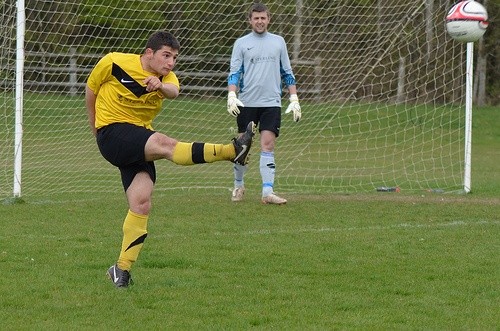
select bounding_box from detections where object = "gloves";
[285,94,302,123]
[226,91,244,117]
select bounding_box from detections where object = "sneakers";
[231,121,258,166]
[231,186,246,201]
[105,263,131,288]
[262,193,287,205]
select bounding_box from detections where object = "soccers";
[446,1,489,41]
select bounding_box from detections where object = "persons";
[85,31,256,287]
[226,3,302,204]
